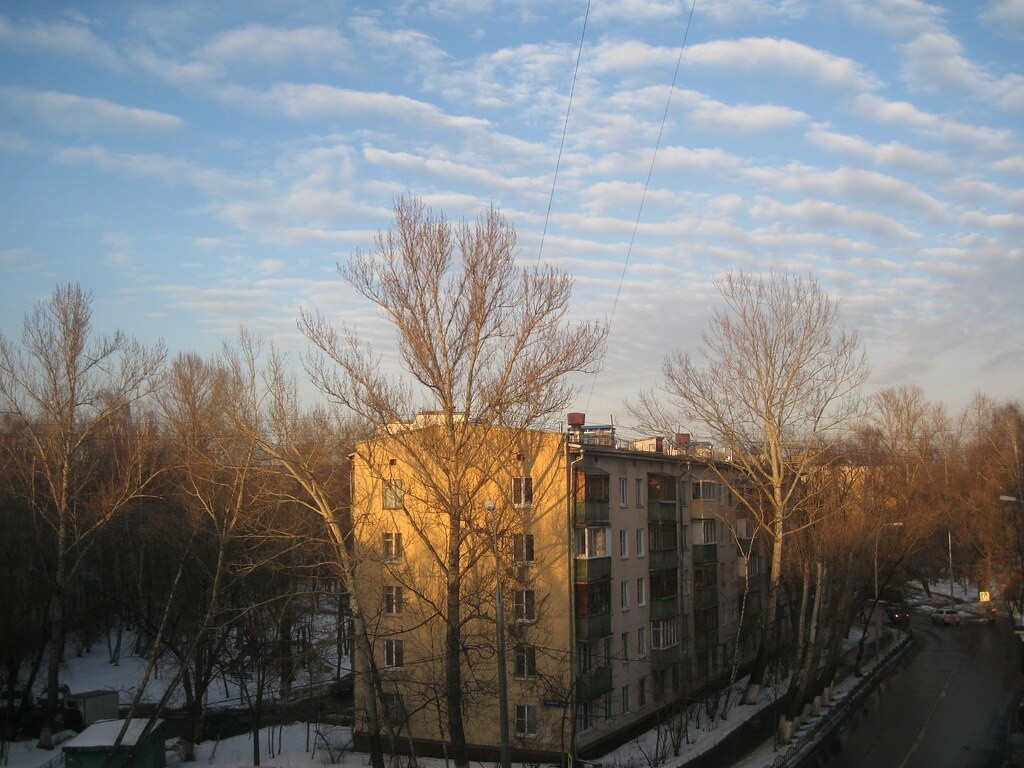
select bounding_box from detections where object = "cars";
[0,686,33,709]
[931,610,960,624]
[882,608,909,624]
[35,684,71,709]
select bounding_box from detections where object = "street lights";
[484,499,511,768]
[875,522,903,662]
